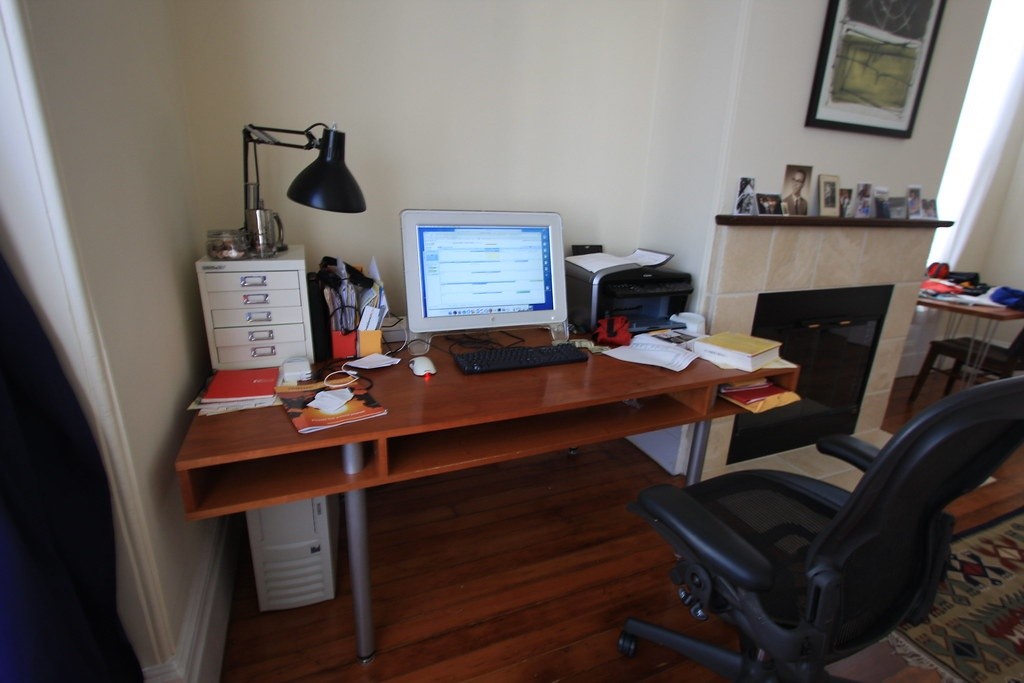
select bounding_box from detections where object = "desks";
[917,297,1024,394]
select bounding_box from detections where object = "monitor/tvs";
[399,208,569,356]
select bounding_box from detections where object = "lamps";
[243,122,368,249]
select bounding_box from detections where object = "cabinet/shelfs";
[175,327,799,666]
[196,246,314,371]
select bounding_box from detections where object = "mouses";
[408,356,436,376]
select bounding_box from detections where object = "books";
[718,376,800,414]
[187,365,389,435]
[645,328,782,373]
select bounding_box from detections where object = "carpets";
[886,508,1024,683]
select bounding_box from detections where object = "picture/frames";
[805,0,947,140]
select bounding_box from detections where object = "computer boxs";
[246,493,339,612]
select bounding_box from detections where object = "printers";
[564,248,693,335]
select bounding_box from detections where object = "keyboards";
[455,344,589,375]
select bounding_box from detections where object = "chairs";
[908,323,1024,408]
[617,377,1024,682]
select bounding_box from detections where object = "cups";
[246,209,283,260]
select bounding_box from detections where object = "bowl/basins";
[207,231,251,260]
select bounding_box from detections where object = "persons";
[0,239,150,683]
[735,164,938,222]
